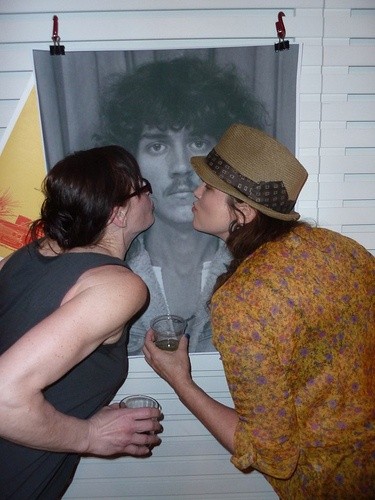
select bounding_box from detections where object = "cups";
[152,315,189,351]
[120,395,161,435]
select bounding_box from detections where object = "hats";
[190,123,309,222]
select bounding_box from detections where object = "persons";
[91,56,273,357]
[0,146,163,500]
[142,123,375,500]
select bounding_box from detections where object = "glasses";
[118,178,154,202]
